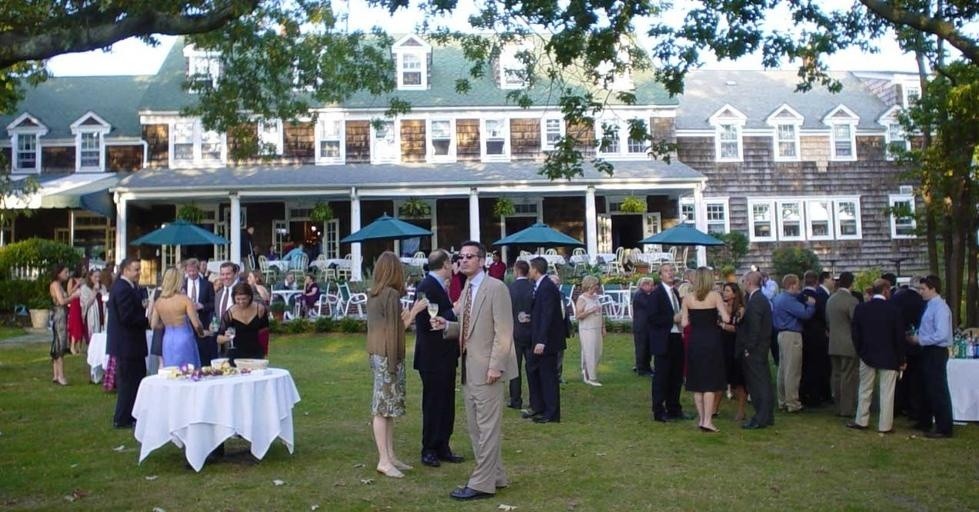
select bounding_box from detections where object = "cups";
[211,317,221,330]
[416,292,427,303]
[190,296,196,305]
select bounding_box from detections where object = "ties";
[190,280,197,305]
[445,287,451,305]
[461,283,473,353]
[670,288,684,332]
[220,288,229,323]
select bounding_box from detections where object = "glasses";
[457,253,480,260]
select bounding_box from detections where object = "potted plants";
[28,293,52,329]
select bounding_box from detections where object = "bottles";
[952,330,979,358]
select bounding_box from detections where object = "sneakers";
[734,414,775,430]
[112,418,136,429]
[521,407,561,424]
[560,377,568,385]
[52,377,71,387]
[923,430,955,441]
[847,422,869,430]
[507,401,522,409]
[69,347,82,356]
[633,364,655,377]
[712,410,721,418]
[654,411,694,423]
[905,421,933,432]
[778,405,804,416]
[879,426,898,434]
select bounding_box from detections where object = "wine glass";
[227,328,237,350]
[426,303,440,331]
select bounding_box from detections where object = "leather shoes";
[421,454,442,468]
[376,462,405,478]
[439,448,465,463]
[450,485,507,501]
[389,457,414,472]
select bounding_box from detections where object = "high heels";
[699,423,721,433]
[581,368,587,383]
[586,379,604,387]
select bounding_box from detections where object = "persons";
[48,256,320,429]
[366,251,430,480]
[634,265,954,439]
[573,276,606,387]
[427,241,520,498]
[240,226,322,271]
[413,247,464,467]
[444,249,572,424]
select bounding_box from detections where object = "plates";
[157,358,269,376]
